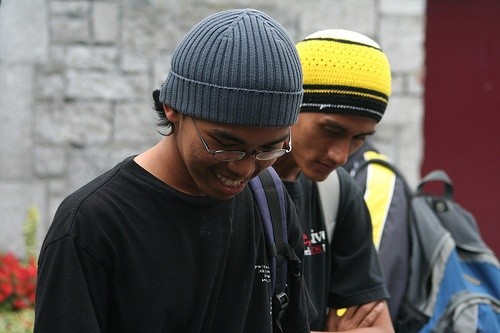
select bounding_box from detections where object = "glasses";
[191,116,292,162]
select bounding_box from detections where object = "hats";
[295,29,392,122]
[159,8,303,126]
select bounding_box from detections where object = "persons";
[30,8,320,332]
[268,27,396,332]
[342,139,410,331]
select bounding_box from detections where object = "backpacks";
[397,167,499,333]
